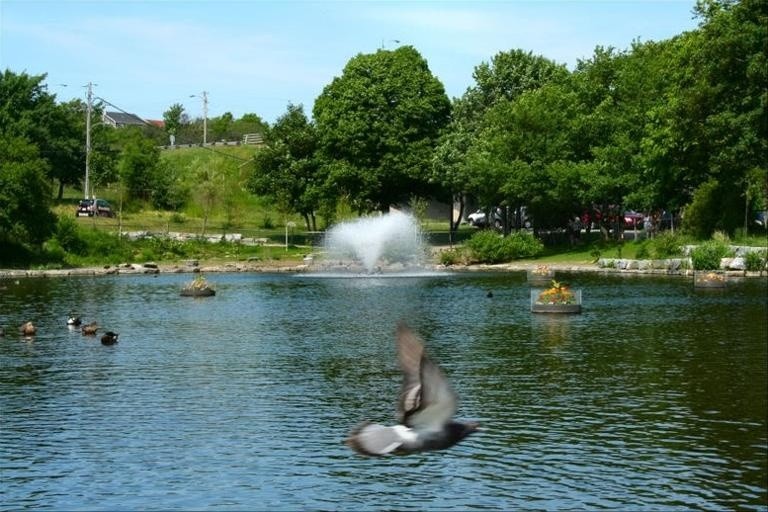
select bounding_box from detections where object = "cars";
[75,198,117,220]
[466,202,645,231]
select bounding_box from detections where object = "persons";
[565,207,678,248]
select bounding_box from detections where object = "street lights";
[189,90,208,143]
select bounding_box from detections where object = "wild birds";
[344,318,490,456]
[101,332,120,347]
[81,322,100,335]
[66,313,83,326]
[18,321,39,338]
